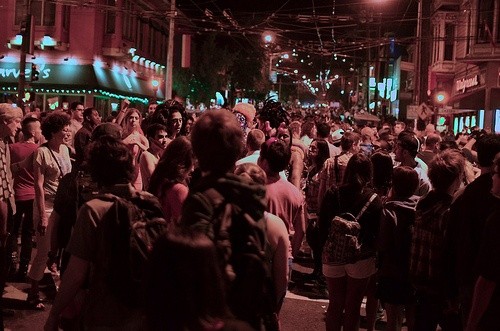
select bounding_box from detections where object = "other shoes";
[375,321,402,331]
[297,251,311,258]
[312,282,330,299]
[25,297,46,310]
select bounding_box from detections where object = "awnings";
[0,62,164,103]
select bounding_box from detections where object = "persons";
[252,141,307,293]
[0,88,499,331]
[313,154,388,331]
[172,109,290,331]
[39,138,172,331]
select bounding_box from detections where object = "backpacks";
[91,191,168,307]
[322,193,378,262]
[204,188,279,320]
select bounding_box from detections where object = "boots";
[8,257,18,282]
[14,260,31,282]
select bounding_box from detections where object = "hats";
[231,102,256,128]
